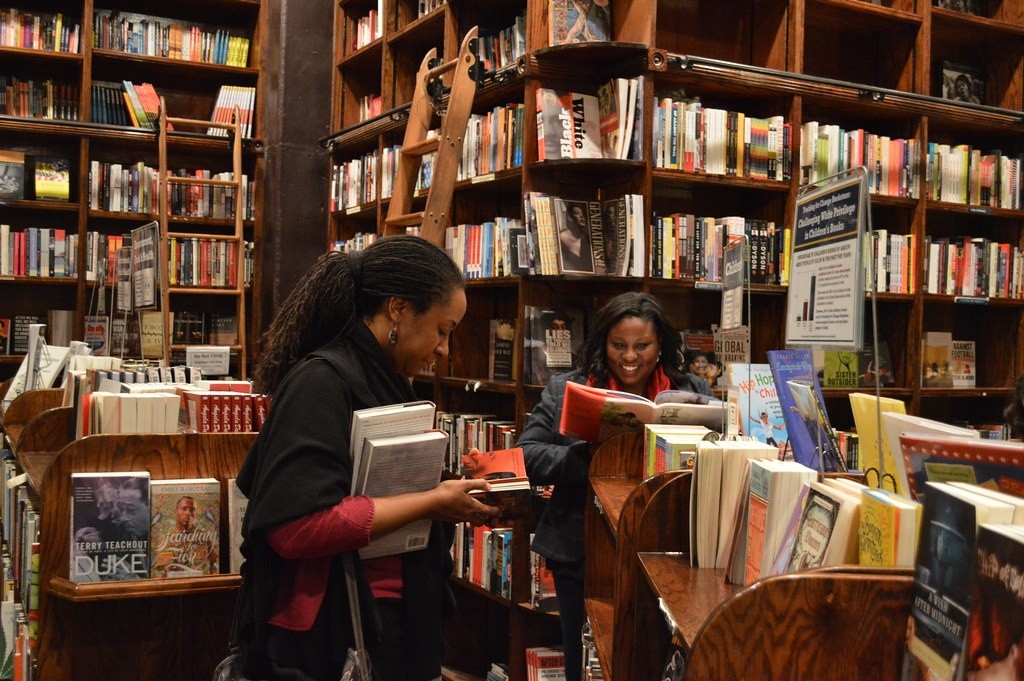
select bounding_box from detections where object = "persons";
[151,494,217,578]
[963,364,971,373]
[703,360,726,389]
[691,352,712,377]
[0,321,7,354]
[76,478,150,580]
[951,74,981,105]
[230,233,503,681]
[518,294,716,681]
[560,206,593,271]
[604,205,617,273]
[750,412,788,448]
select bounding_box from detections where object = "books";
[228,479,249,575]
[348,400,437,499]
[0,6,274,681]
[355,427,450,562]
[558,377,732,442]
[151,478,221,578]
[330,0,1024,680]
[70,472,152,582]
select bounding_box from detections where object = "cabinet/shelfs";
[0,361,277,681]
[0,0,279,384]
[322,0,1024,681]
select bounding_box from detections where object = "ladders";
[159,97,246,383]
[380,24,482,384]
